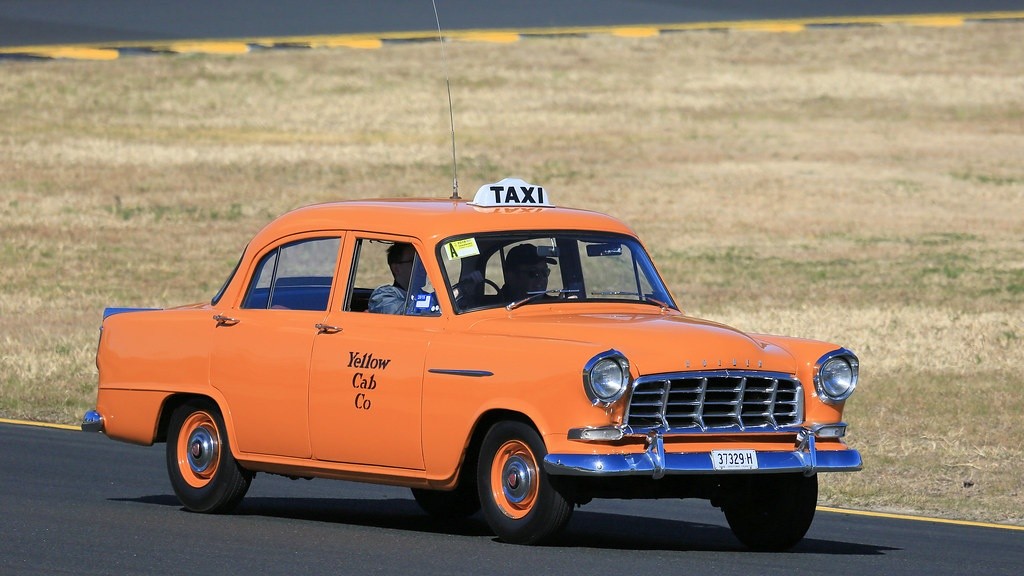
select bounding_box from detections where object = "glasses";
[393,259,422,269]
[512,267,551,278]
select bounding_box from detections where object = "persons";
[493,242,559,304]
[367,242,485,316]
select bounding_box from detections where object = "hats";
[505,244,557,271]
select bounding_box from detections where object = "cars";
[81,177,865,552]
